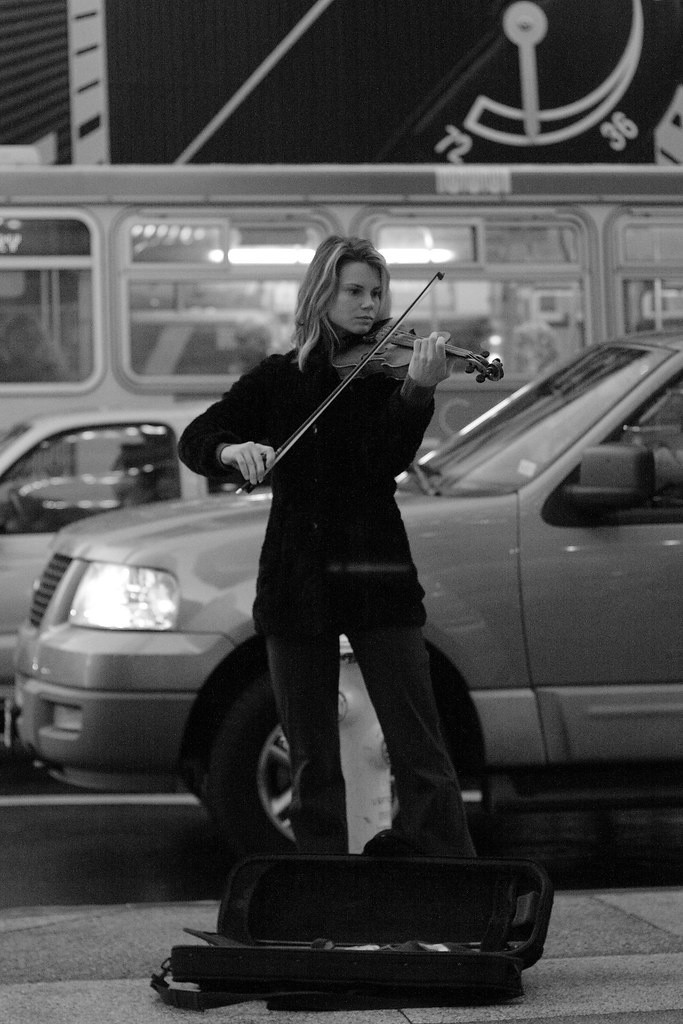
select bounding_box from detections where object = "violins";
[332,316,505,383]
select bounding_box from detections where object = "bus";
[1,141,682,442]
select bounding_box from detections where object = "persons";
[176,234,478,854]
[0,311,70,381]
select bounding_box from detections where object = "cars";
[0,399,231,691]
[4,331,682,865]
